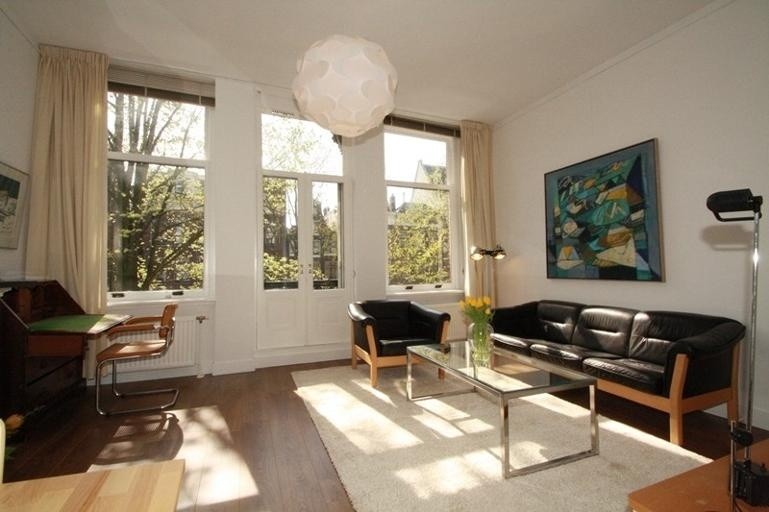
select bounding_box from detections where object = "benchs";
[489,297,747,446]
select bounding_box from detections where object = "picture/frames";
[0,160,34,251]
[542,138,666,283]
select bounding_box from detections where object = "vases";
[466,320,495,360]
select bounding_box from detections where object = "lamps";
[466,241,507,298]
[290,23,403,140]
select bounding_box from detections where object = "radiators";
[85,309,209,383]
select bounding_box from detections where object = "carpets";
[290,358,715,511]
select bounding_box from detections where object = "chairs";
[347,298,452,389]
[95,302,180,416]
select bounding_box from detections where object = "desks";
[0,279,132,440]
[0,458,188,511]
[405,339,599,480]
[625,436,769,511]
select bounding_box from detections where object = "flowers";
[457,293,496,346]
